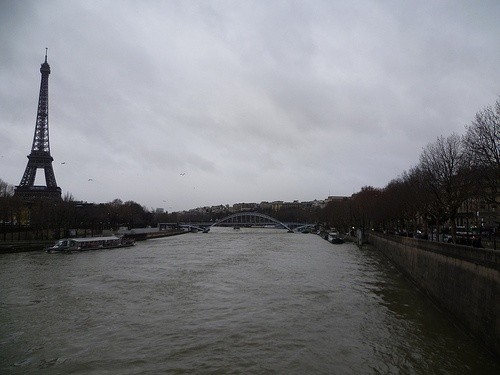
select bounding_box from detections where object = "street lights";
[491,205,497,249]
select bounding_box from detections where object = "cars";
[394,226,480,246]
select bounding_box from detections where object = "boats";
[298,224,345,244]
[49,235,137,252]
[233,225,240,230]
